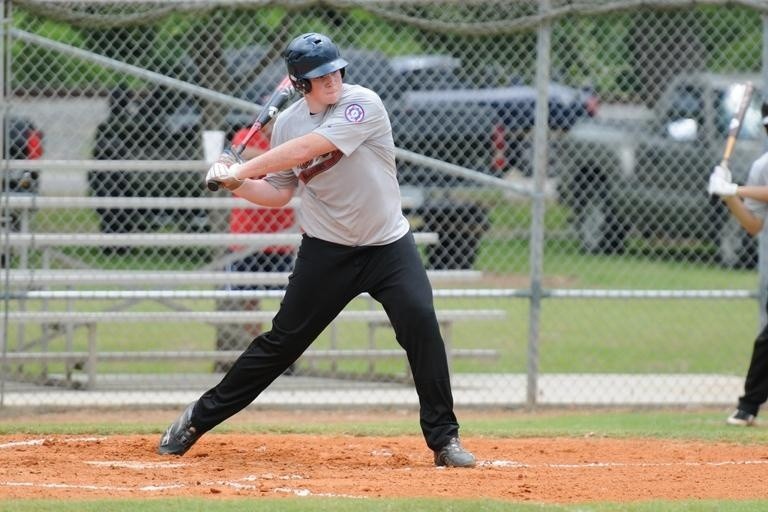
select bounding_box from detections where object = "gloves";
[206,145,245,188]
[709,164,737,197]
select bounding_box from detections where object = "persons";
[707,102,768,426]
[230,124,301,377]
[159,32,476,468]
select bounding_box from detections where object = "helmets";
[285,32,349,94]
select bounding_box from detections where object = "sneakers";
[726,408,754,425]
[159,402,201,456]
[434,437,477,466]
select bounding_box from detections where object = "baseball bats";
[207,73,295,191]
[709,82,755,205]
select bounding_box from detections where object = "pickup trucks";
[556,71,763,269]
